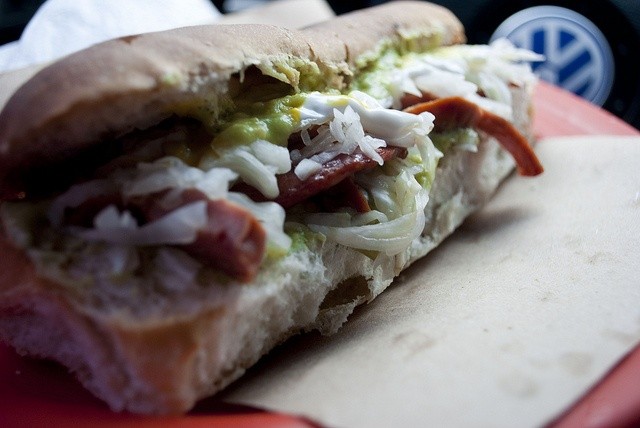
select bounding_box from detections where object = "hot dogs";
[1,1,546,415]
